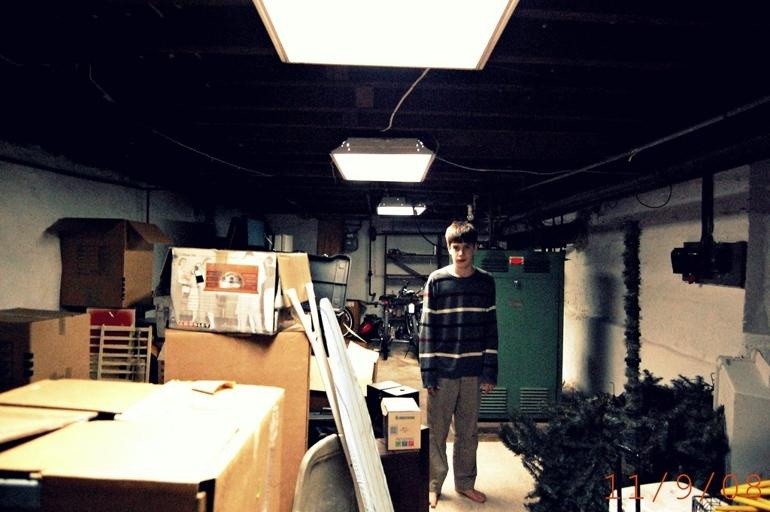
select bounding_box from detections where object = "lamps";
[330,137,434,182]
[254,1,520,71]
[378,197,427,216]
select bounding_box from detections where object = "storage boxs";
[0,216,423,511]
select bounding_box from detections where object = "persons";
[418,219,499,508]
[173,251,273,333]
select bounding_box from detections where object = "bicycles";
[394,280,425,363]
[374,290,400,363]
[331,308,355,339]
